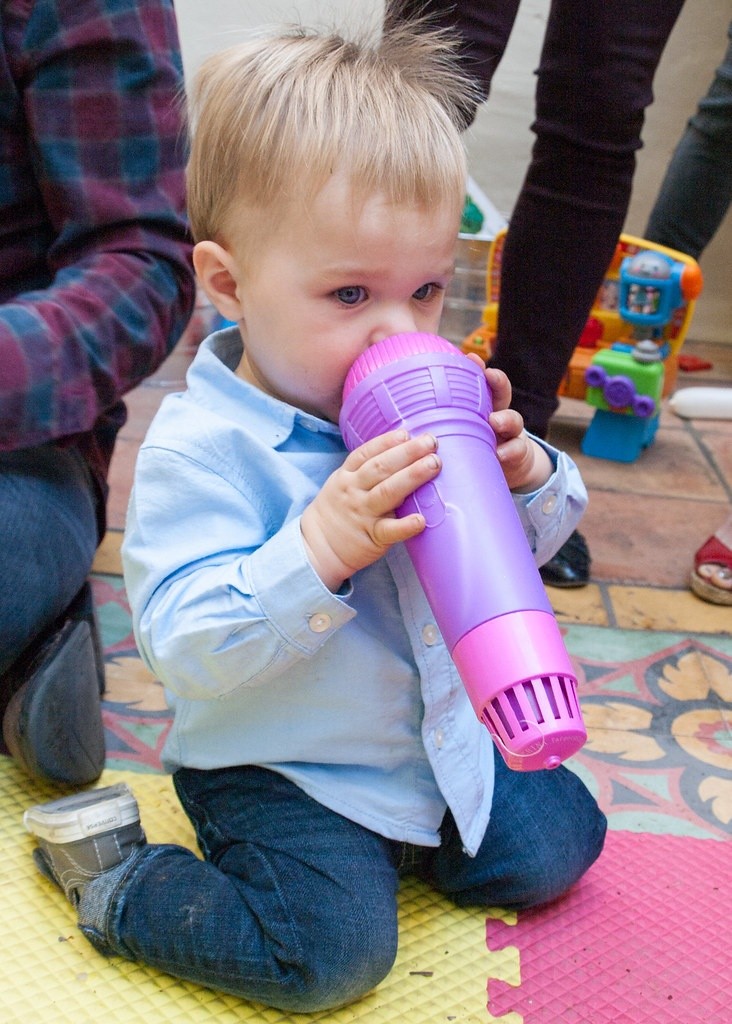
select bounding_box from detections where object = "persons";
[384,0,732,607]
[25,33,609,1014]
[0,1,195,791]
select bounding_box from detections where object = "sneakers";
[23,784,141,914]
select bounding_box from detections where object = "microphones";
[337,332,588,774]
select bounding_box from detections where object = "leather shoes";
[540,528,591,589]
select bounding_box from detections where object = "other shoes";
[4,584,108,787]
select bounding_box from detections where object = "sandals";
[691,534,732,606]
